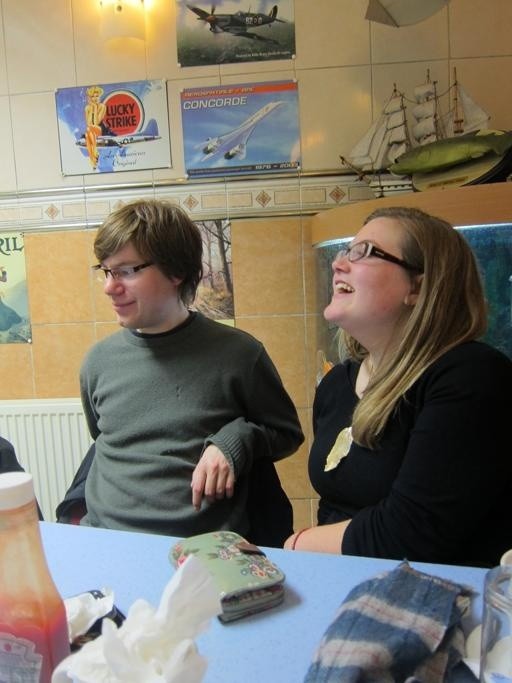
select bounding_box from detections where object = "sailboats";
[340,65,494,195]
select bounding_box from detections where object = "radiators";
[0,397,95,519]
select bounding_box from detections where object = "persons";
[283,206,511,567]
[79,199,305,547]
[83,86,107,173]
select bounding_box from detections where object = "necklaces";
[322,425,354,472]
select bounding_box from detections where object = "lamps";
[96,1,150,48]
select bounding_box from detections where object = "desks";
[36,520,512,683]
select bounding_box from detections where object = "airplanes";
[193,98,289,162]
[73,115,161,148]
[186,5,286,47]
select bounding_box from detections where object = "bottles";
[1,470,70,683]
[481,547,511,682]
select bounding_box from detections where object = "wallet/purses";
[168,531,286,625]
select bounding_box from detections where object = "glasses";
[90,263,156,281]
[335,242,425,277]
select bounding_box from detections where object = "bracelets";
[292,526,309,550]
